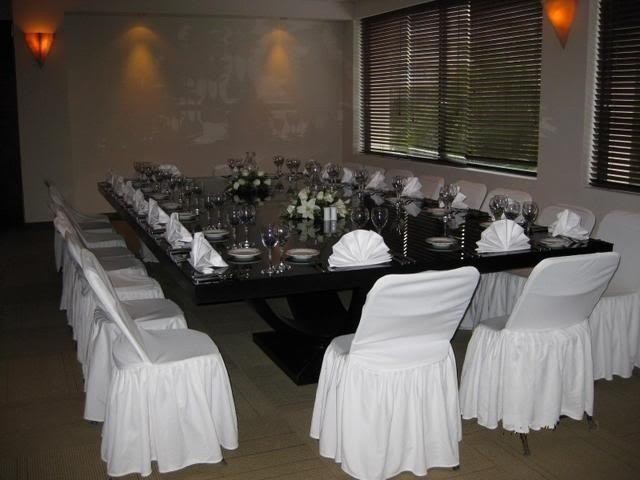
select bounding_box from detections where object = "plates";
[228,247,263,260]
[426,236,459,247]
[134,179,230,241]
[428,207,448,215]
[540,237,569,248]
[285,247,322,262]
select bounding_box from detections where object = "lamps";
[23,33,54,68]
[542,1,577,50]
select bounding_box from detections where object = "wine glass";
[260,224,292,275]
[133,160,257,249]
[272,151,408,207]
[484,195,538,236]
[439,183,457,218]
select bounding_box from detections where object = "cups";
[349,207,389,233]
